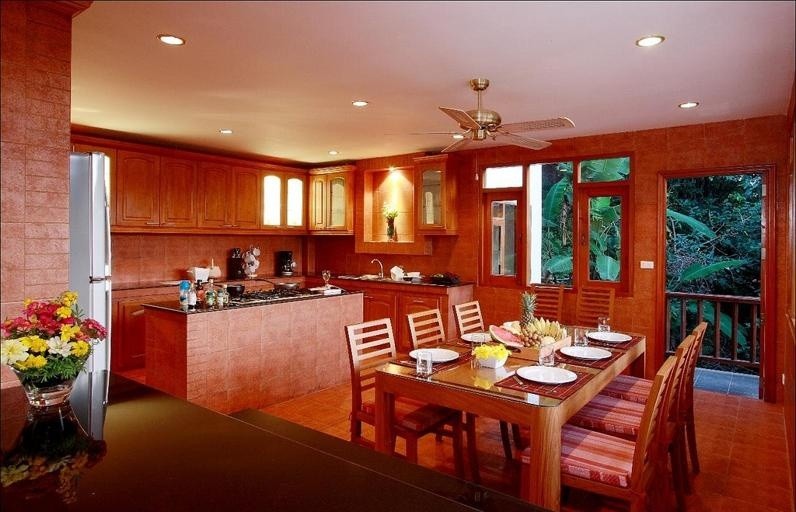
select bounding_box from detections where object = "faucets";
[370,258,384,279]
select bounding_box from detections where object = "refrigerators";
[70,151,113,440]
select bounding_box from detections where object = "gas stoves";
[228,288,321,305]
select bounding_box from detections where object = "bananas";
[521,317,564,348]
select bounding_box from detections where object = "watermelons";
[488,324,525,350]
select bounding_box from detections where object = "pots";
[255,278,300,290]
[213,283,245,293]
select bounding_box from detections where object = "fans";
[407,78,577,155]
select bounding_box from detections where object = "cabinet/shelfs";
[262,164,309,235]
[308,165,355,235]
[412,154,459,234]
[116,141,196,233]
[72,135,116,235]
[197,153,261,235]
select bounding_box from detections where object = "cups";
[415,335,485,375]
[539,317,610,367]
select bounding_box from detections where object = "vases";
[22,400,88,436]
[13,369,80,408]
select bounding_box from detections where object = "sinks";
[360,274,386,281]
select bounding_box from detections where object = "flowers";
[0,289,109,384]
[0,416,108,506]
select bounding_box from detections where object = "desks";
[1,369,487,512]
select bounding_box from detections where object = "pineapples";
[519,291,538,324]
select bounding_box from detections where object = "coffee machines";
[277,250,293,276]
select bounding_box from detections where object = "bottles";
[178,278,229,309]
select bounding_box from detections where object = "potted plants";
[381,201,399,243]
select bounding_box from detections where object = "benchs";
[226,407,557,512]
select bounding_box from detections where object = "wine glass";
[322,271,331,286]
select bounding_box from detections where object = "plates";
[515,332,632,385]
[408,321,516,364]
